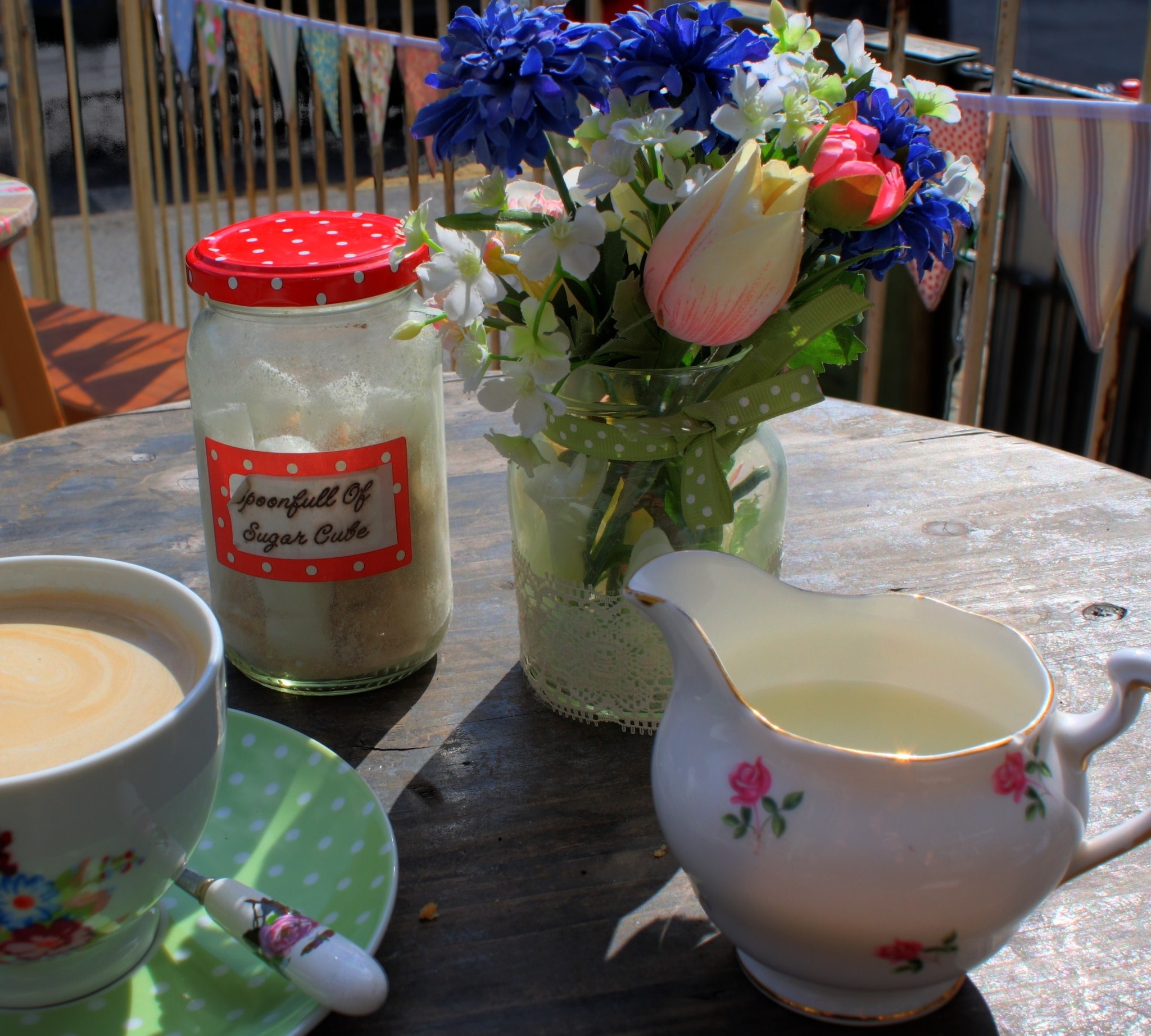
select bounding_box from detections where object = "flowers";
[411,1,985,609]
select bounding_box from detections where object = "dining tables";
[0,367,1150,1036]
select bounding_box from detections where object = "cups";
[629,547,1148,1025]
[1,556,224,1005]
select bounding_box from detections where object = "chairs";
[0,224,192,448]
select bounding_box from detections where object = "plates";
[0,703,402,1033]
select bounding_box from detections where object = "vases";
[503,395,792,737]
